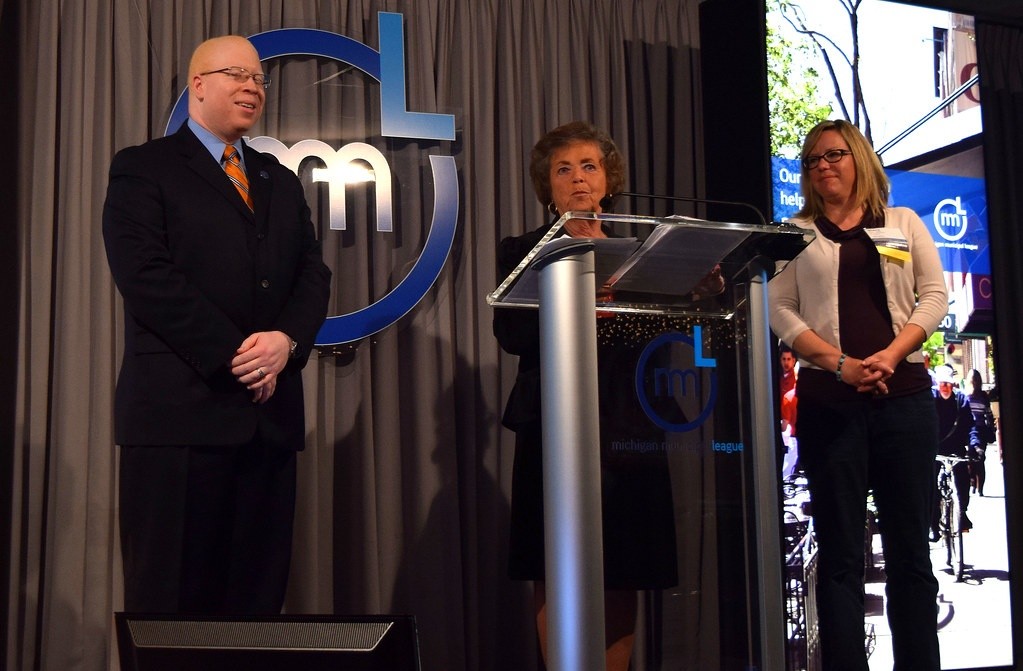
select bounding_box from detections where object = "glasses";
[802,148,853,170]
[198,65,272,89]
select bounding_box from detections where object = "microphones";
[600,192,765,225]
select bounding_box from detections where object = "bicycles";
[936,451,981,583]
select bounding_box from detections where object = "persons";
[774,314,1004,551]
[100,34,335,624]
[493,118,726,671]
[766,116,949,668]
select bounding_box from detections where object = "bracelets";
[835,351,847,383]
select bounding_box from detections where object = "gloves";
[970,446,985,463]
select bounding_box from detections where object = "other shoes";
[960,512,973,530]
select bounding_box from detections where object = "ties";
[223,145,253,212]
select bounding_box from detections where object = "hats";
[936,365,954,383]
